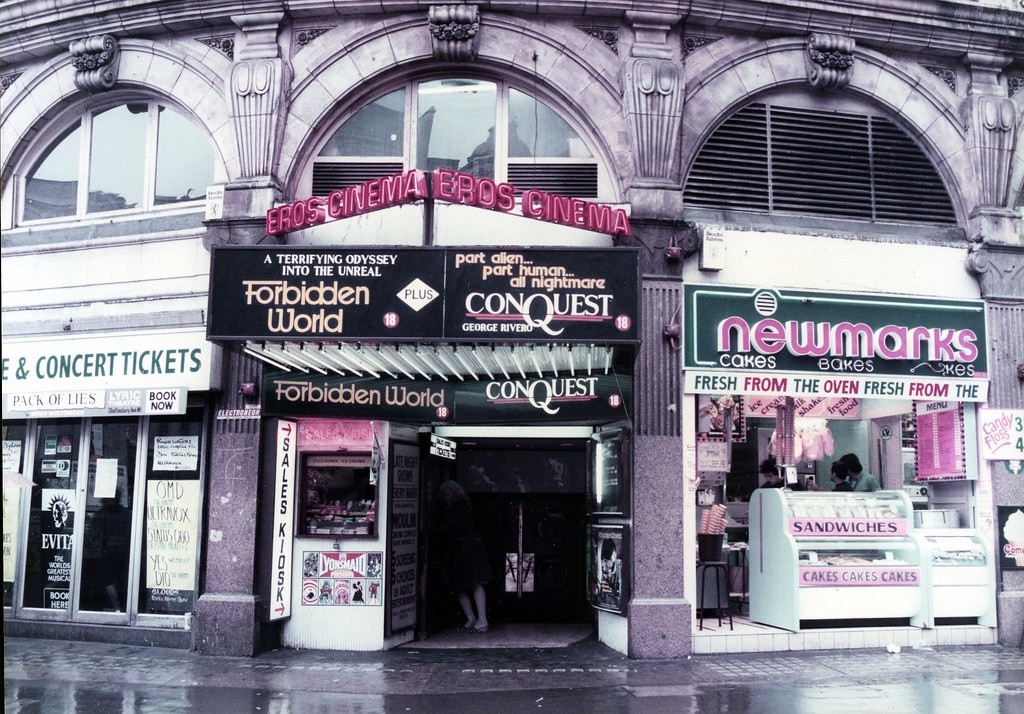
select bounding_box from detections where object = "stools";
[695,560,733,630]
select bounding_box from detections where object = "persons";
[89,485,142,613]
[438,480,488,633]
[758,453,880,492]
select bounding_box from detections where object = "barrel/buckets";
[698,533,723,561]
[914,509,960,528]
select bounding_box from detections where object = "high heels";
[469,623,488,633]
[457,621,477,633]
